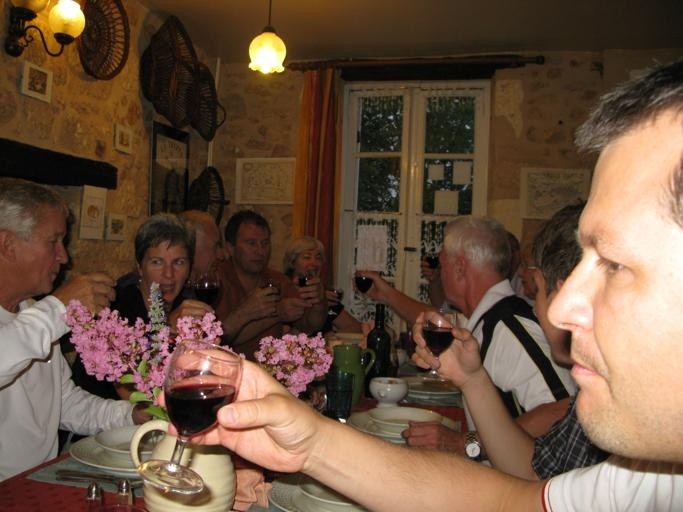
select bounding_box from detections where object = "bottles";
[366,302,392,400]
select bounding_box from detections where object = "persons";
[281,236,364,336]
[112,210,281,348]
[156,54,683,512]
[1,177,154,482]
[213,209,327,364]
[517,225,538,301]
[352,214,577,462]
[70,212,216,402]
[421,231,536,314]
[412,198,613,481]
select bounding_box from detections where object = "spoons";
[56,467,144,489]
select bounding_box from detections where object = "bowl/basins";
[369,376,405,409]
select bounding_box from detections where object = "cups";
[328,370,352,419]
[330,345,374,407]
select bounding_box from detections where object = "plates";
[297,474,357,506]
[347,412,458,445]
[97,425,164,459]
[267,474,370,512]
[68,434,146,479]
[402,377,458,399]
[366,407,442,433]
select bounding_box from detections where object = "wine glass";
[326,289,342,318]
[195,272,217,307]
[421,309,457,379]
[260,277,279,301]
[297,267,314,292]
[136,339,242,494]
[353,271,372,305]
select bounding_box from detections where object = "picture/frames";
[18,63,54,104]
[234,158,294,203]
[520,167,591,219]
[147,119,191,216]
[114,122,132,155]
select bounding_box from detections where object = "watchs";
[463,430,483,462]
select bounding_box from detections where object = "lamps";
[5,1,86,58]
[248,0,288,75]
